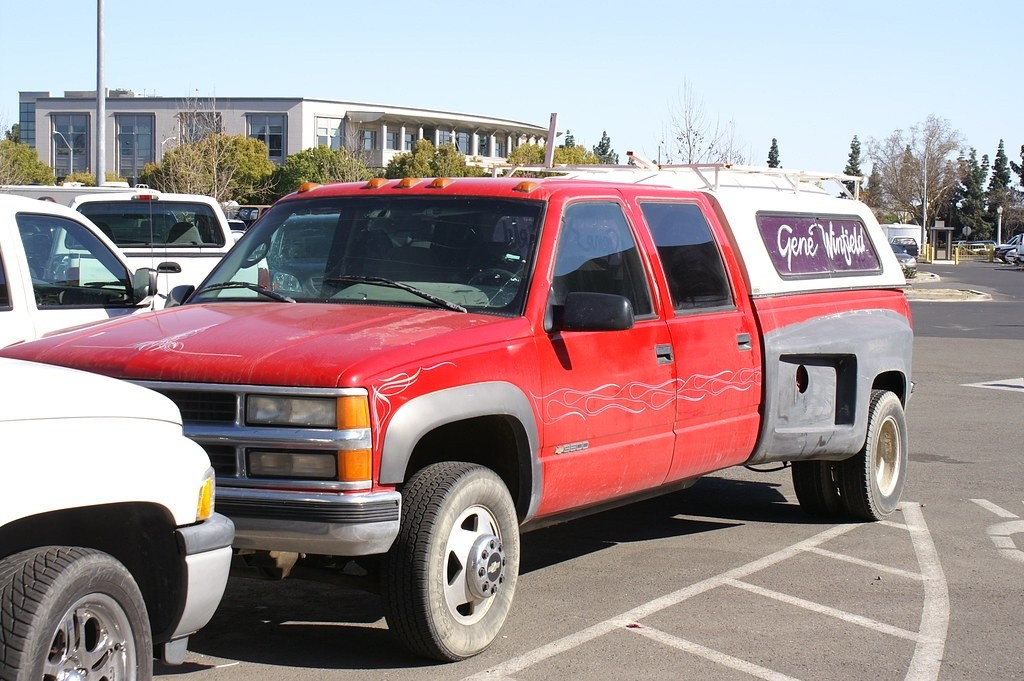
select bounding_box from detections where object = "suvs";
[889,236,920,259]
[890,242,916,278]
[0,179,380,352]
[951,233,1024,267]
[1,349,237,681]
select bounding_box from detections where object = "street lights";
[161,137,177,192]
[996,205,1003,245]
[51,130,73,175]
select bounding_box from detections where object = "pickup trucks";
[1,113,917,662]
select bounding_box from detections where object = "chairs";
[430,215,488,281]
[346,229,393,276]
[95,222,116,242]
[166,222,195,243]
